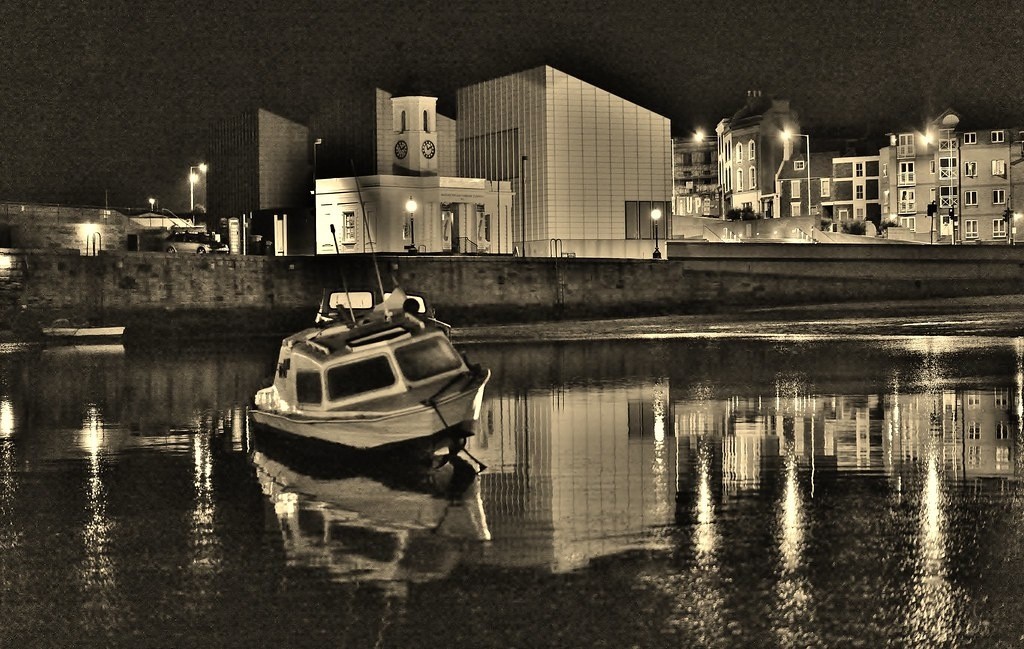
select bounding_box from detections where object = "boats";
[245,435,496,649]
[246,155,492,468]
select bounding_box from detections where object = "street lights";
[405,194,418,256]
[189,164,208,214]
[650,208,664,258]
[780,131,812,217]
[695,132,726,220]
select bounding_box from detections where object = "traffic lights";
[1008,209,1014,220]
[1003,209,1009,223]
[949,207,954,217]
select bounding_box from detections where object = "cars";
[243,234,272,255]
[161,231,230,257]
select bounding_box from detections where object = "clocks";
[394,140,408,159]
[422,139,436,160]
